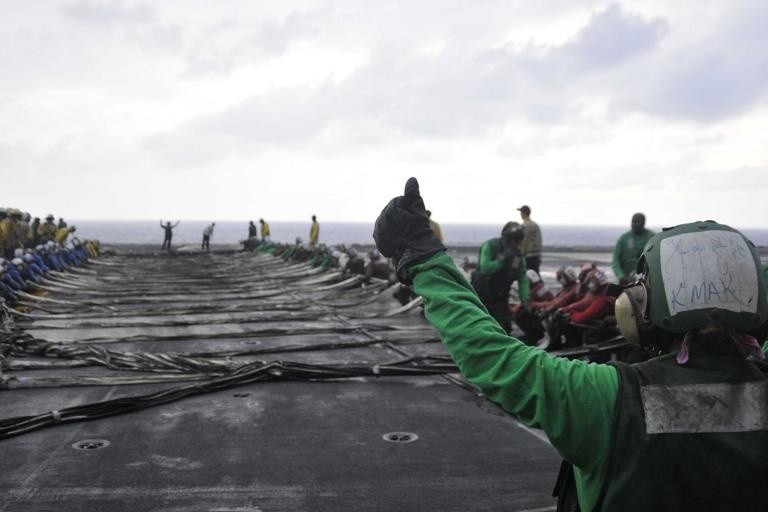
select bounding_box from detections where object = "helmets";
[0,207,53,221]
[526,263,607,292]
[635,221,768,354]
[502,223,524,239]
[0,236,83,273]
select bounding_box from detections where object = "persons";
[247,221,256,240]
[259,218,270,243]
[425,209,443,242]
[202,223,216,251]
[159,218,180,248]
[0,209,78,263]
[470,205,658,361]
[365,249,415,306]
[307,216,321,245]
[370,176,768,512]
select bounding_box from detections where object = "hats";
[517,205,531,215]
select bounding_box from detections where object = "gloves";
[504,246,521,258]
[373,177,447,285]
